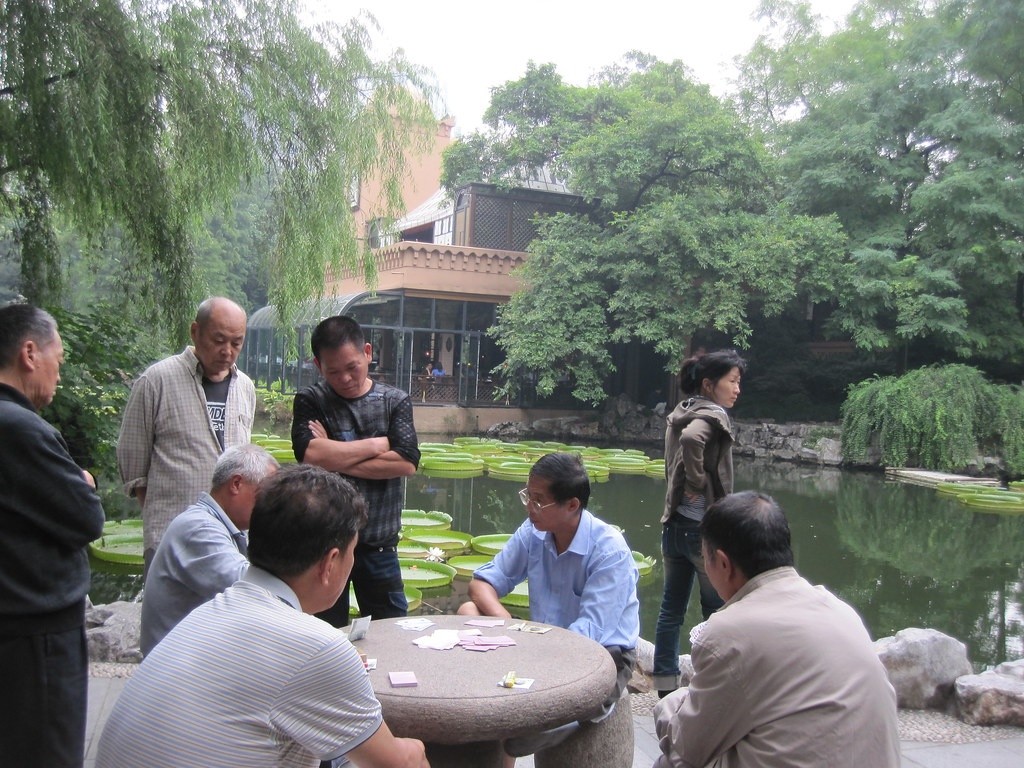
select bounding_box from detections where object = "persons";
[91,443,431,768]
[290,314,421,630]
[801,299,816,342]
[0,303,104,768]
[654,349,742,699]
[458,451,639,768]
[654,489,901,768]
[420,362,445,382]
[117,297,257,578]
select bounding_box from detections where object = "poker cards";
[464,618,506,629]
[396,617,432,633]
[412,627,517,652]
[387,670,418,688]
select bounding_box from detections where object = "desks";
[338,614,617,768]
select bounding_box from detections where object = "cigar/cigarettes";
[519,620,528,630]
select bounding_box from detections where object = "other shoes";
[658,689,677,698]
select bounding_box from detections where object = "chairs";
[431,375,453,400]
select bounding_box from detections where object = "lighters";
[504,671,517,687]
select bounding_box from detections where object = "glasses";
[519,488,556,514]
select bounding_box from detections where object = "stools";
[534,686,634,768]
